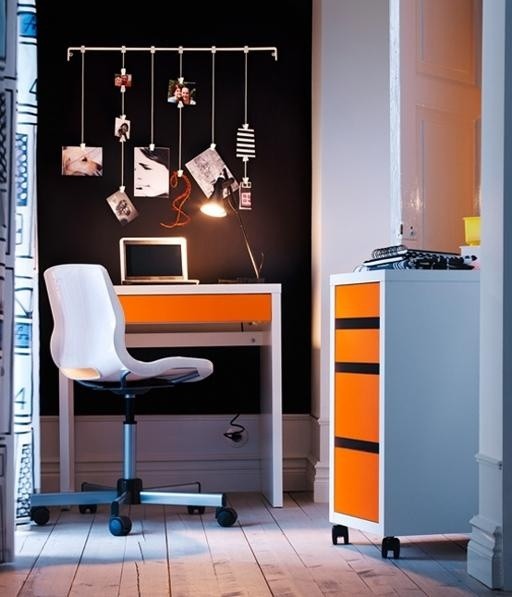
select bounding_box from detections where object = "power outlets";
[227,427,247,447]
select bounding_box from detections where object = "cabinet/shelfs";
[331,272,481,559]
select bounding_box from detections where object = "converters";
[231,434,241,441]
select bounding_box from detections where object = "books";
[362,245,474,272]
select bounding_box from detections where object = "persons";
[168,81,193,103]
[121,75,131,86]
[115,75,122,86]
[135,148,169,197]
[113,199,127,216]
[182,87,196,105]
[117,123,128,139]
[118,205,132,224]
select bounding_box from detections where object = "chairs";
[29,265,237,539]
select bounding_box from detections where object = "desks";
[59,282,282,514]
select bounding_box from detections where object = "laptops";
[119,237,200,285]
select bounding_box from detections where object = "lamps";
[201,170,265,283]
